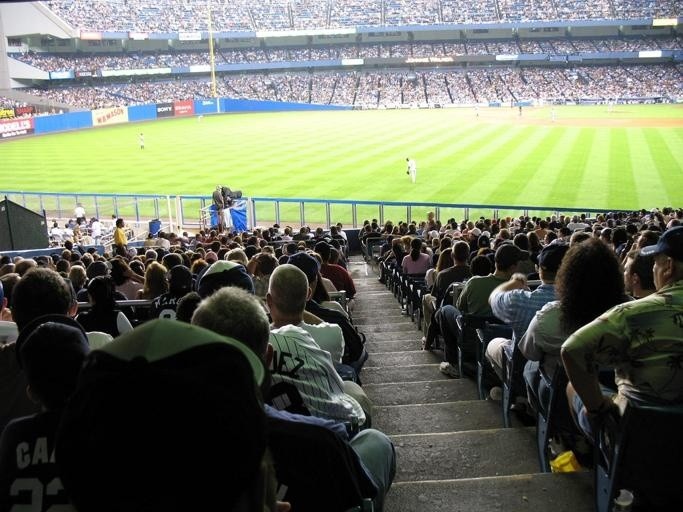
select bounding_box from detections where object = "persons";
[0,184,394,512]
[358,204,682,512]
[405,158,415,183]
[139,133,144,150]
[0,0,682,116]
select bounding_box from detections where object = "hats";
[197,260,254,298]
[495,244,569,269]
[429,230,439,238]
[640,226,683,255]
[287,253,321,281]
[468,228,481,236]
[15,314,88,407]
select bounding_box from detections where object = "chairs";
[359,236,544,387]
[55,343,380,512]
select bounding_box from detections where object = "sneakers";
[440,362,463,378]
